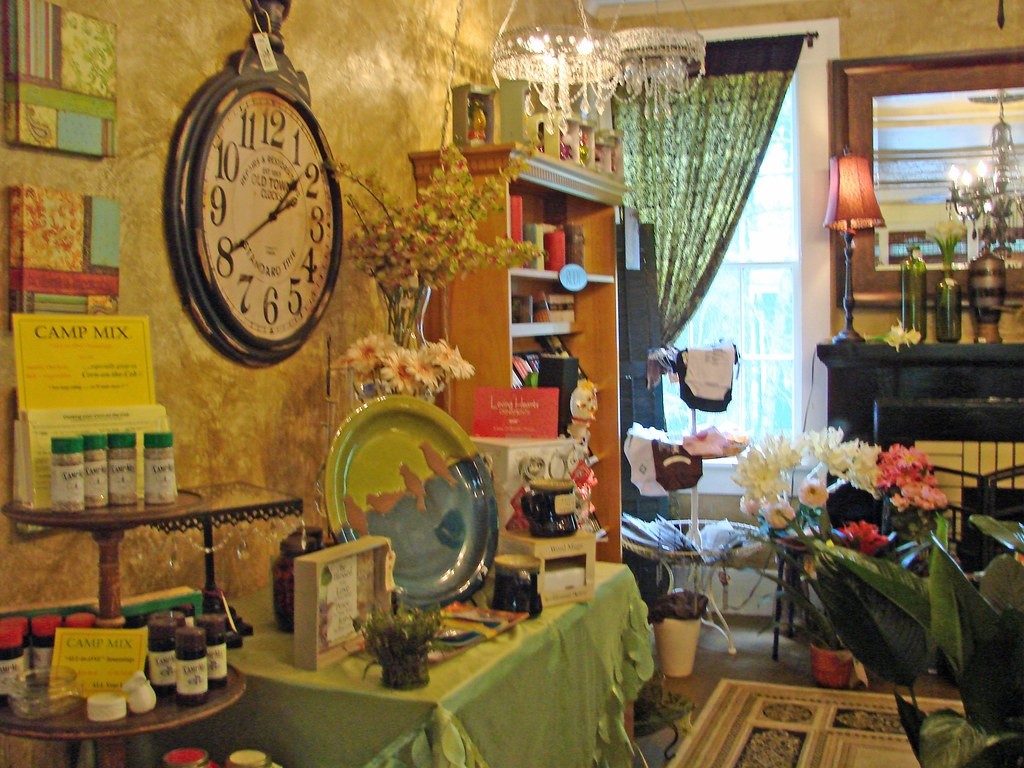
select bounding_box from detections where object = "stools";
[769,535,823,660]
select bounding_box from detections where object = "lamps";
[943,84,1024,247]
[489,1,705,136]
[823,146,885,344]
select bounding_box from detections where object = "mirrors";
[826,46,1024,310]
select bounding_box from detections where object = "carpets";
[665,678,966,768]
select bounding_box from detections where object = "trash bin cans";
[651,593,707,678]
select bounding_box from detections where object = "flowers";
[314,147,546,406]
[869,317,922,352]
[732,426,948,653]
[930,213,969,302]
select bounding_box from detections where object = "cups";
[491,553,545,620]
[521,478,579,538]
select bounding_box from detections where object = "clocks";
[162,0,343,370]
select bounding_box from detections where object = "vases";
[809,642,854,688]
[935,267,964,342]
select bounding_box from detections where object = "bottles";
[146,602,228,707]
[899,247,927,343]
[52,430,177,514]
[0,612,96,706]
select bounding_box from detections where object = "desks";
[81,561,654,768]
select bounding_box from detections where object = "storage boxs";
[472,433,587,531]
[452,83,498,146]
[500,525,595,605]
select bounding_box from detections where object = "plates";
[324,393,501,611]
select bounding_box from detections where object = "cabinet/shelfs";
[407,142,629,565]
[0,490,246,768]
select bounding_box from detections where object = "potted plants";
[349,596,443,692]
